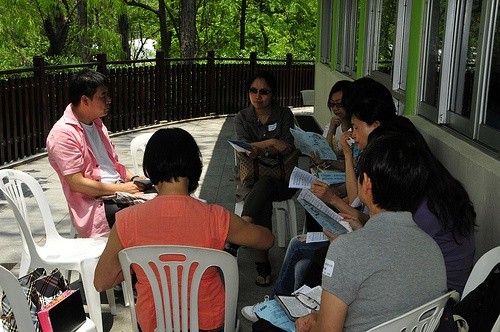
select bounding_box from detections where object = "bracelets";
[130,175,139,181]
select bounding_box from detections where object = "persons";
[93,127,275,332]
[238,78,477,324]
[251,133,446,332]
[219,73,299,287]
[44,68,156,239]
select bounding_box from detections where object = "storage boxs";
[38,288,87,332]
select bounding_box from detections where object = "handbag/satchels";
[237,149,300,185]
[1,267,68,332]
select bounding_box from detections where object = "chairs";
[365,290,457,332]
[0,168,117,332]
[0,265,97,332]
[456,246,500,332]
[130,133,154,178]
[234,149,297,247]
[119,245,240,332]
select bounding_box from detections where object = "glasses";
[329,102,343,108]
[294,292,320,312]
[250,86,272,95]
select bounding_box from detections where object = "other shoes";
[241,304,261,322]
[254,261,274,287]
